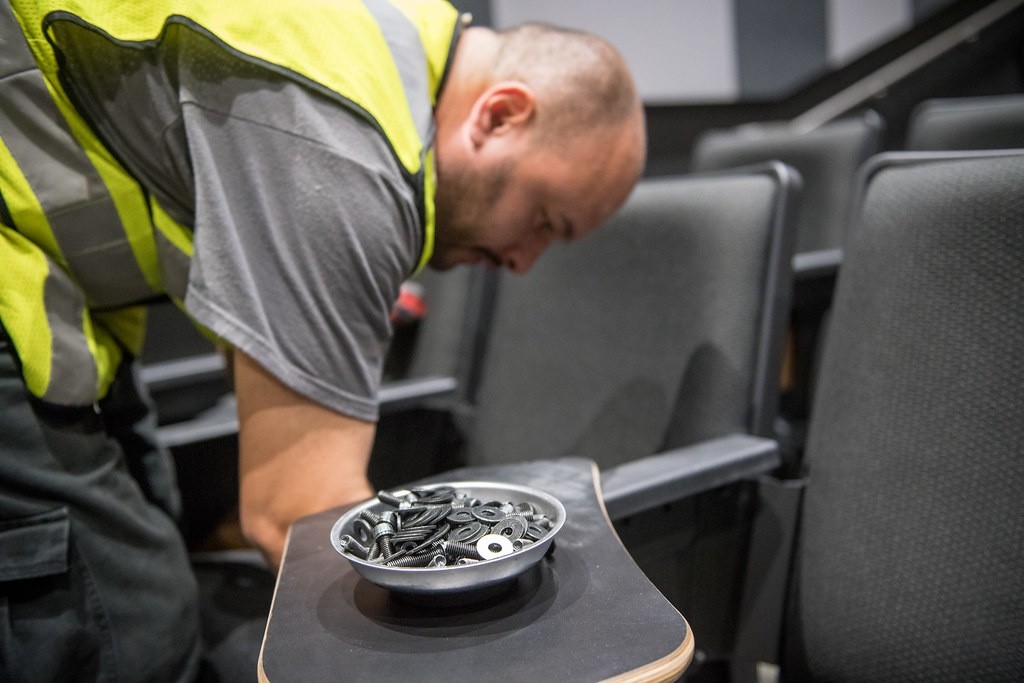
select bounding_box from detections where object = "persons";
[0,0,649,682]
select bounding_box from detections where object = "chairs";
[140,89,1023,682]
